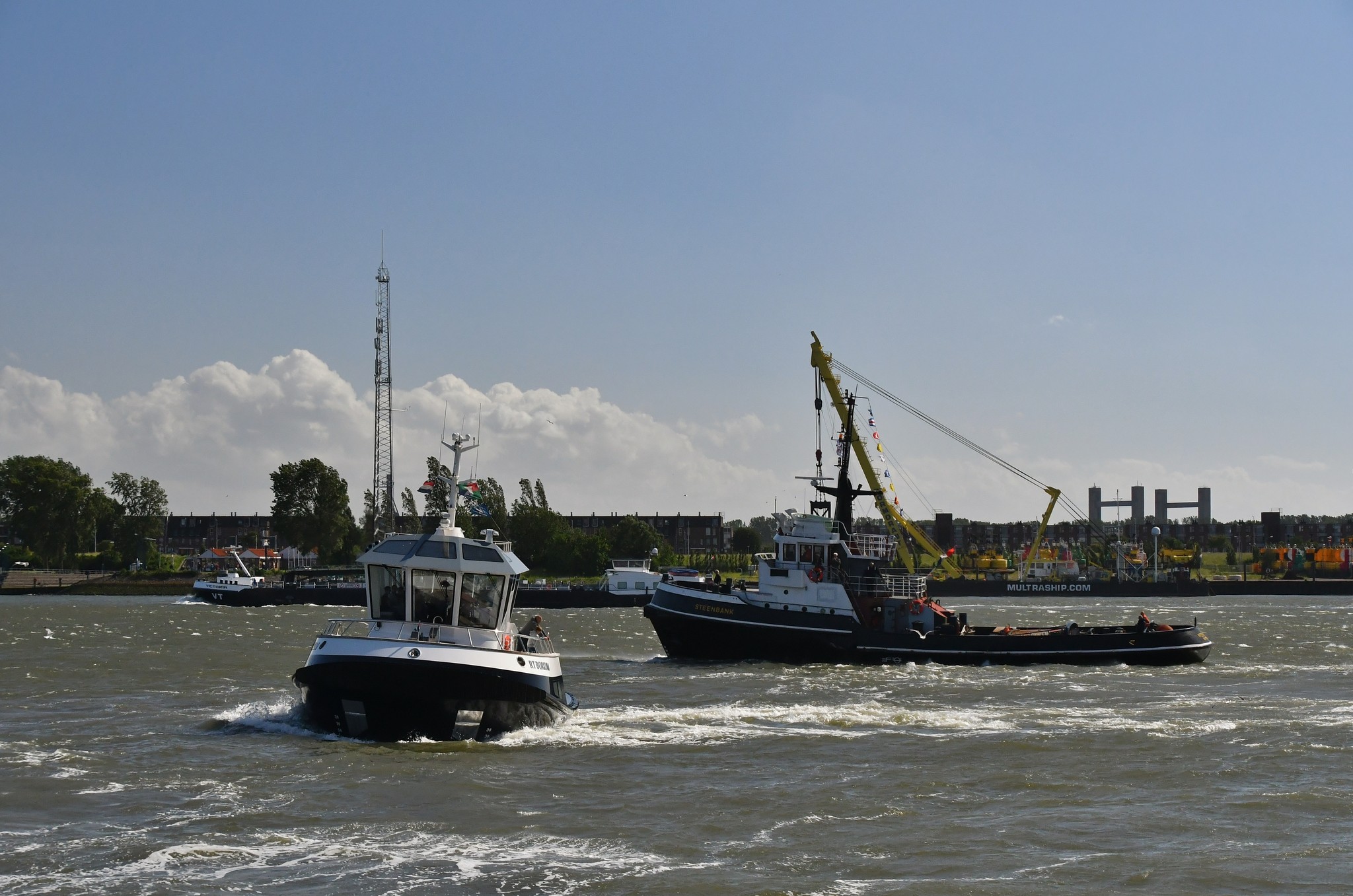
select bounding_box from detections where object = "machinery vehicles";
[804,329,1061,581]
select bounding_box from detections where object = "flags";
[868,408,904,518]
[417,481,436,495]
[469,502,491,518]
[836,441,844,457]
[455,482,484,502]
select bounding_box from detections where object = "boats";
[604,559,706,608]
[643,383,1215,667]
[887,578,1210,597]
[193,548,265,607]
[290,399,579,744]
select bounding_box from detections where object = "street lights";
[1012,549,1026,582]
[1151,526,1161,582]
[1022,526,1025,549]
[1030,525,1033,545]
[1116,540,1121,579]
[1252,516,1255,547]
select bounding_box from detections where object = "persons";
[803,545,823,564]
[380,585,406,621]
[516,615,551,652]
[210,563,219,571]
[527,626,548,653]
[831,552,841,584]
[863,561,883,598]
[714,569,721,585]
[1134,611,1150,633]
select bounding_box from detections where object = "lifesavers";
[909,600,923,615]
[505,636,510,650]
[546,584,552,590]
[809,567,823,582]
